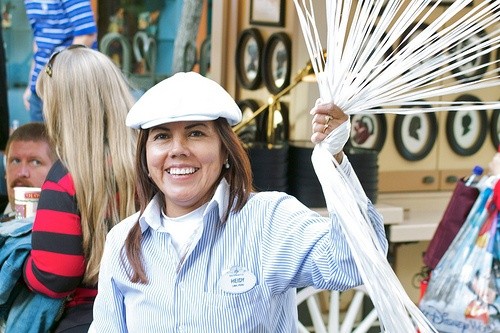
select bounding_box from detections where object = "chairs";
[296,284,378,333]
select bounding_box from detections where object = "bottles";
[460,165,484,189]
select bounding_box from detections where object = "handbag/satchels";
[415,174,500,332]
[423,177,479,269]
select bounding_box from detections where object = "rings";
[323,116,333,133]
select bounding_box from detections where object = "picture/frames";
[249,0,286,27]
[235,28,292,145]
[393,99,439,161]
[446,94,488,156]
[349,0,500,88]
[490,98,500,152]
[346,106,387,154]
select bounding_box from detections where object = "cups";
[13,186,42,221]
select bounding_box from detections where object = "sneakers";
[125,72,245,129]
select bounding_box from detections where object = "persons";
[86,72,388,333]
[0,1,136,333]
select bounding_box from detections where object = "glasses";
[46,43,86,77]
